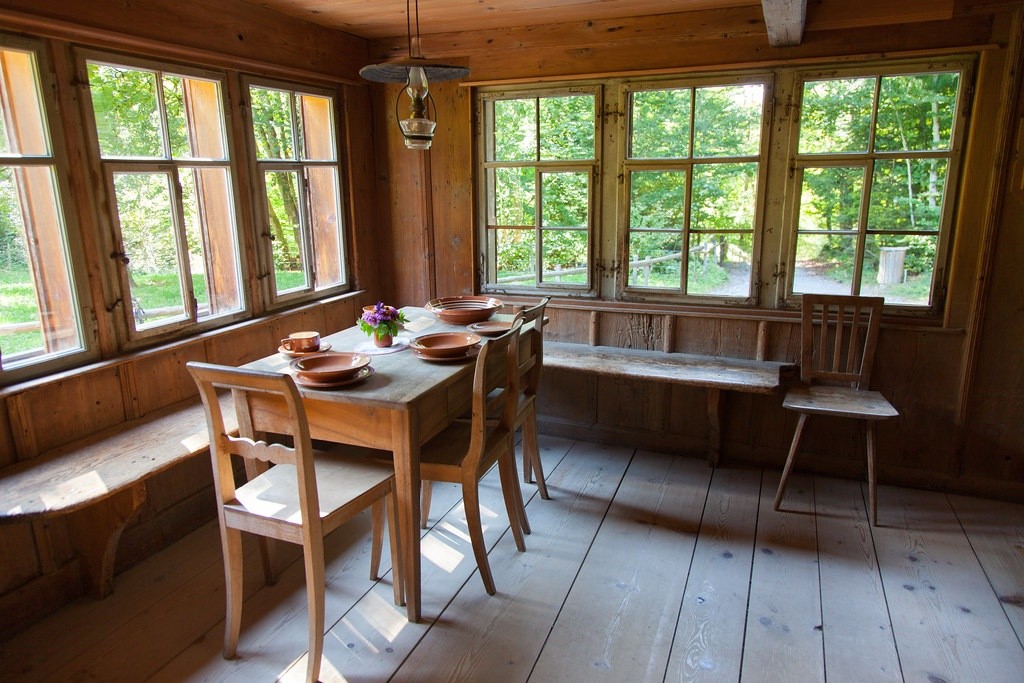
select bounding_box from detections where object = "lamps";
[358,0,469,150]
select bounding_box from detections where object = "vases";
[374,329,393,348]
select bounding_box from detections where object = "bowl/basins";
[289,352,371,382]
[425,296,502,324]
[410,332,481,357]
[363,305,395,314]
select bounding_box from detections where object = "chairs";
[185,360,404,683]
[771,294,899,526]
[456,296,551,535]
[370,317,527,597]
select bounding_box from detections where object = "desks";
[212,306,550,624]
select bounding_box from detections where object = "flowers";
[355,300,411,340]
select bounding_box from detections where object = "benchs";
[541,339,796,472]
[0,387,239,600]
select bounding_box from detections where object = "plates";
[413,344,481,361]
[466,321,513,335]
[290,366,375,386]
[278,344,332,357]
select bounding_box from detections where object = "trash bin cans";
[876,246,910,283]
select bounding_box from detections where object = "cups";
[513,305,525,314]
[281,332,320,352]
